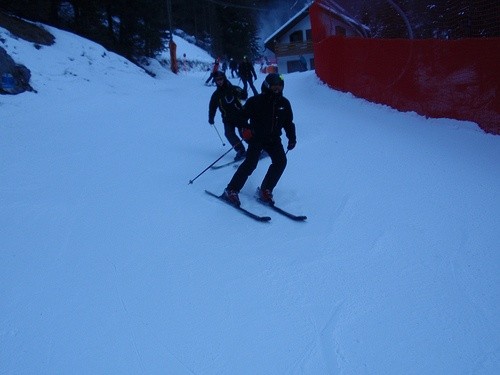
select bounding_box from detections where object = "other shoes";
[258,187,275,206]
[234,150,246,160]
[222,188,241,206]
[259,149,268,159]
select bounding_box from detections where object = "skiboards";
[211,151,264,170]
[205,190,307,221]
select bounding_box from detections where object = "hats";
[261,73,284,96]
[240,56,248,63]
[214,71,226,86]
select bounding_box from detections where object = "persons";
[220,72,297,207]
[206,55,259,163]
[267,63,285,97]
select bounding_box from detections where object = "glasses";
[216,78,224,81]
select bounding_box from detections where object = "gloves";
[209,119,214,124]
[236,88,242,95]
[241,127,254,139]
[287,140,296,150]
